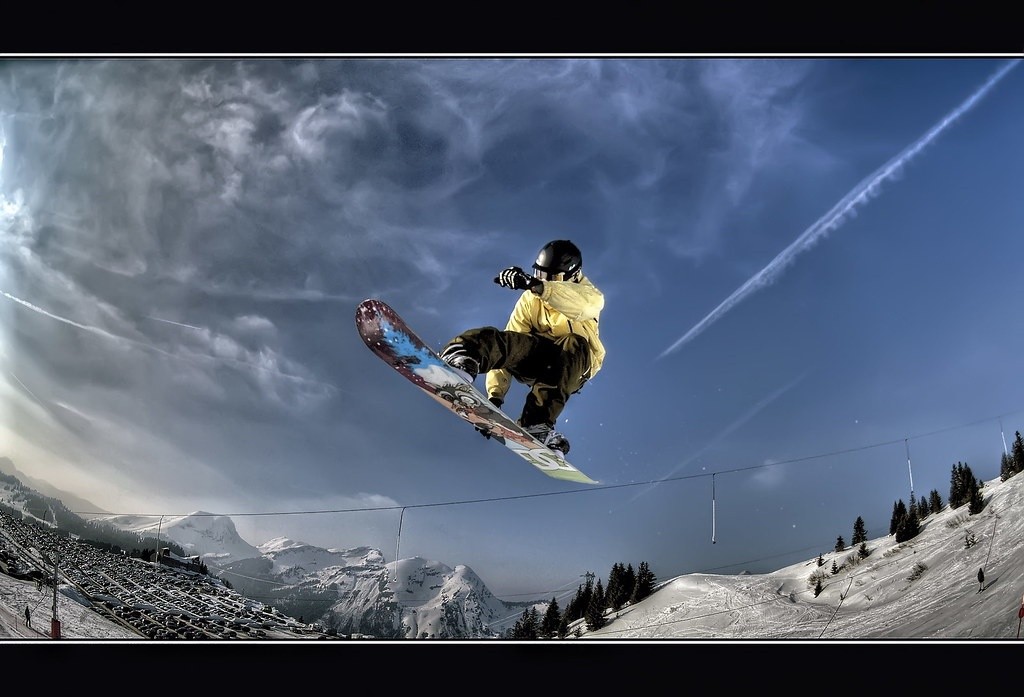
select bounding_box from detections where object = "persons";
[25,606,31,627]
[439,239,607,455]
[978,568,984,589]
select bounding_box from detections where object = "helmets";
[531,239,583,282]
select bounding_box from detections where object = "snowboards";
[355,297,600,486]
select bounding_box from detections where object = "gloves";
[493,266,544,295]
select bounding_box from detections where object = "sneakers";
[516,418,569,456]
[436,342,479,384]
